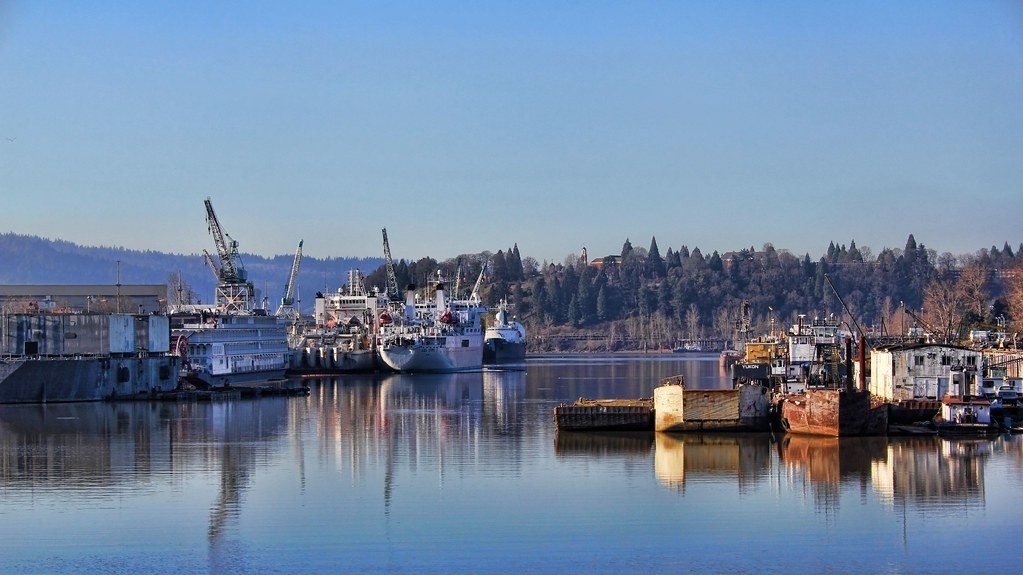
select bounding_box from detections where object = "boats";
[167,195,528,378]
[719,272,860,396]
